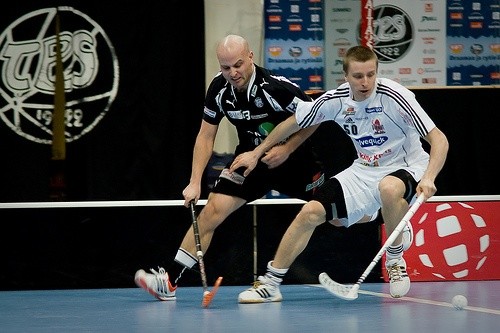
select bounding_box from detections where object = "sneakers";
[135,265,177,300]
[238,281,282,301]
[380,257,410,298]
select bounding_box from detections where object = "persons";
[229,46,449,304]
[134,34,413,301]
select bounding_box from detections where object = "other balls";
[451,294,468,310]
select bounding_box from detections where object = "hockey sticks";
[317,191,425,301]
[188,200,224,309]
